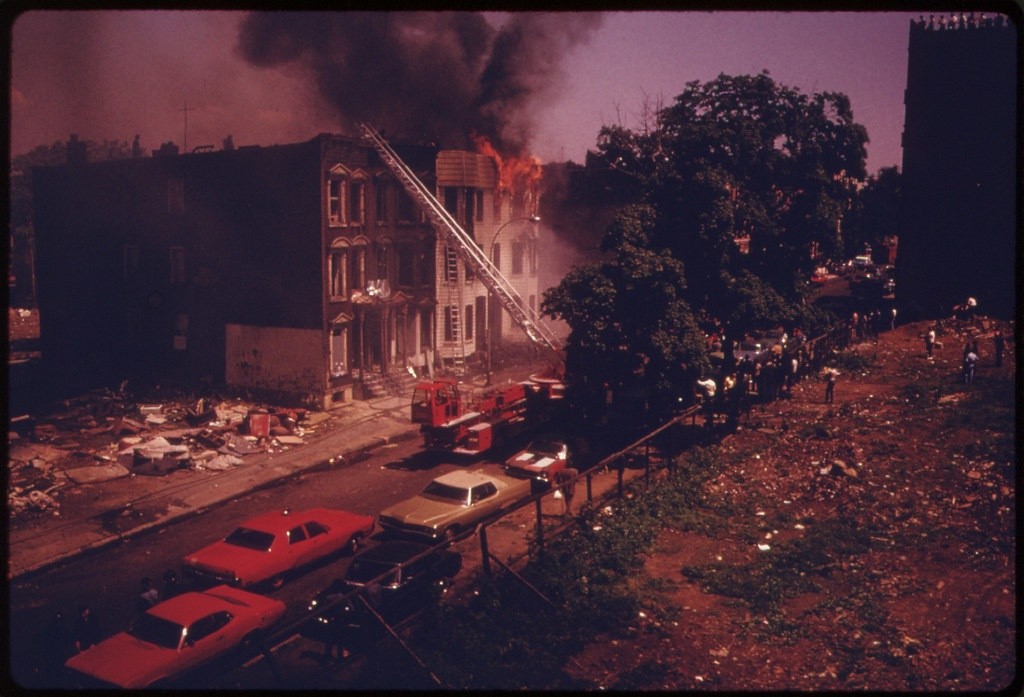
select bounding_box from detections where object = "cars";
[375,468,510,547]
[853,255,875,270]
[179,507,377,594]
[502,435,590,485]
[307,537,462,641]
[64,583,288,692]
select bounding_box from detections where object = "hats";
[164,570,176,580]
[139,576,153,584]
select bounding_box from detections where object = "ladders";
[445,236,466,378]
[354,118,567,366]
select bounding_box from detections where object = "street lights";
[484,216,543,390]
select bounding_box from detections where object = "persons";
[558,460,580,515]
[695,310,811,420]
[994,328,1004,368]
[925,326,935,360]
[852,305,897,343]
[967,295,977,322]
[823,364,837,404]
[964,339,979,382]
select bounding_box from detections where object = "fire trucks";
[352,117,583,462]
